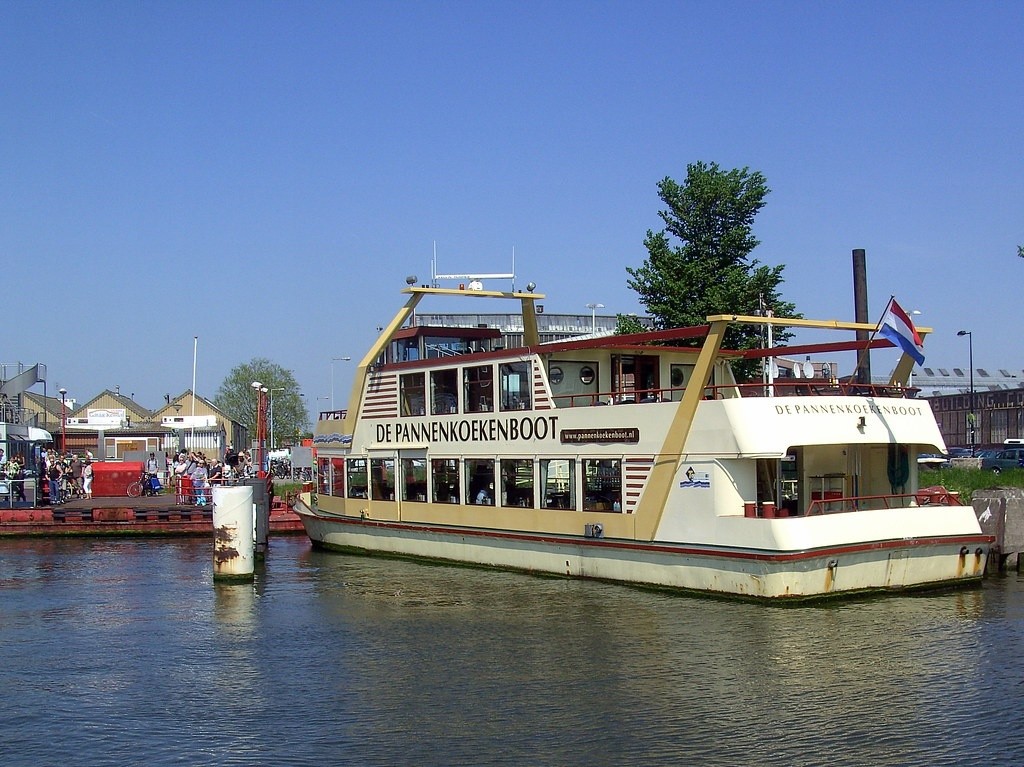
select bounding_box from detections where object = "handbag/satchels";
[151,477,160,491]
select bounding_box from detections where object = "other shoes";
[196,499,201,504]
[61,500,66,504]
[50,501,56,504]
[77,496,79,498]
[80,496,83,500]
[57,501,61,505]
[202,501,207,506]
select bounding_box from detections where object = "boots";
[85,493,91,499]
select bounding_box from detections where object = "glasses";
[180,460,183,461]
[200,463,204,464]
[50,461,54,462]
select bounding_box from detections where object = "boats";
[292,237,997,607]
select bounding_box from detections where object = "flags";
[878,298,926,366]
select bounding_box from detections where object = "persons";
[0,449,26,502]
[86,450,94,459]
[209,459,222,485]
[476,483,488,504]
[36,448,93,505]
[233,452,249,478]
[173,449,210,506]
[145,452,161,496]
[224,446,233,459]
[245,452,251,462]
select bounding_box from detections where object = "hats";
[227,445,233,449]
[238,451,245,456]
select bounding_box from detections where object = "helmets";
[150,452,155,457]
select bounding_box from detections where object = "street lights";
[315,397,329,418]
[270,387,285,450]
[957,330,975,458]
[331,357,350,417]
[250,382,263,478]
[172,405,183,416]
[58,388,68,452]
[261,386,268,438]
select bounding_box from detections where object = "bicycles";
[126,471,154,498]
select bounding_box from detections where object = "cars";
[917,438,1024,476]
